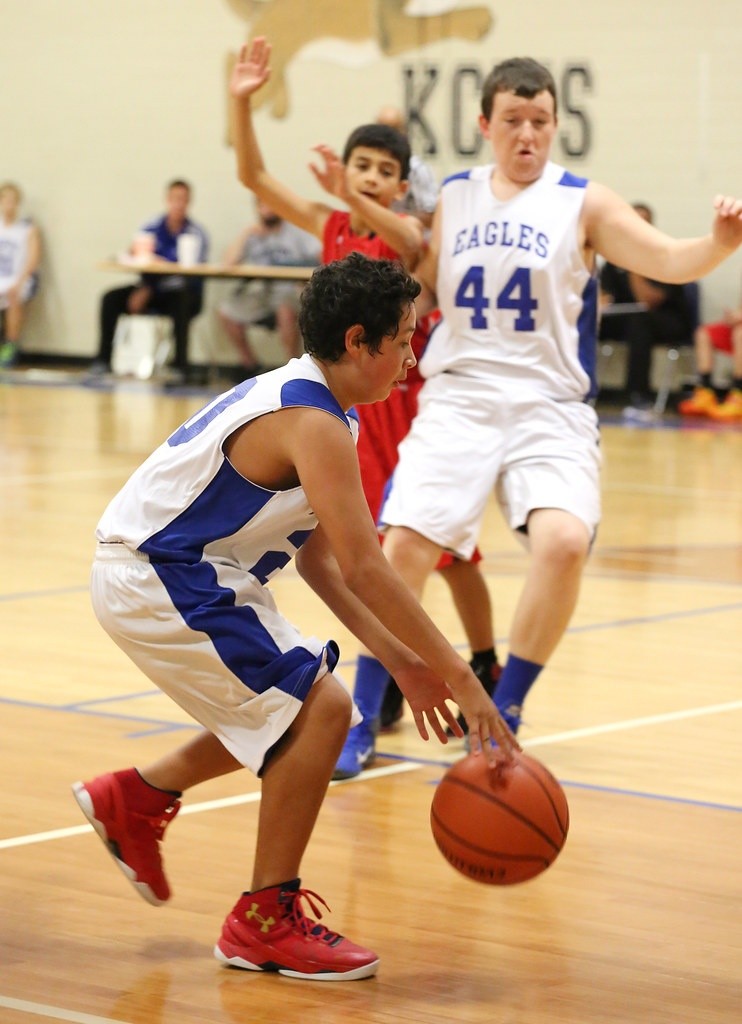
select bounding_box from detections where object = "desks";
[97,263,318,388]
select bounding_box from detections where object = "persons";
[85,181,210,386]
[212,193,322,380]
[375,106,440,245]
[598,201,686,420]
[229,35,503,738]
[74,253,523,982]
[0,184,43,368]
[327,58,742,777]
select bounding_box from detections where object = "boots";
[331,654,388,781]
[465,655,544,750]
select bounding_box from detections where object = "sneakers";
[72,768,182,906]
[214,879,382,982]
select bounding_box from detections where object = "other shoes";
[680,387,742,422]
[156,366,193,383]
[446,659,503,737]
[380,676,404,728]
[78,358,110,373]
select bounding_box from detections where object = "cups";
[176,234,202,264]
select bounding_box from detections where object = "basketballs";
[430,746,570,887]
